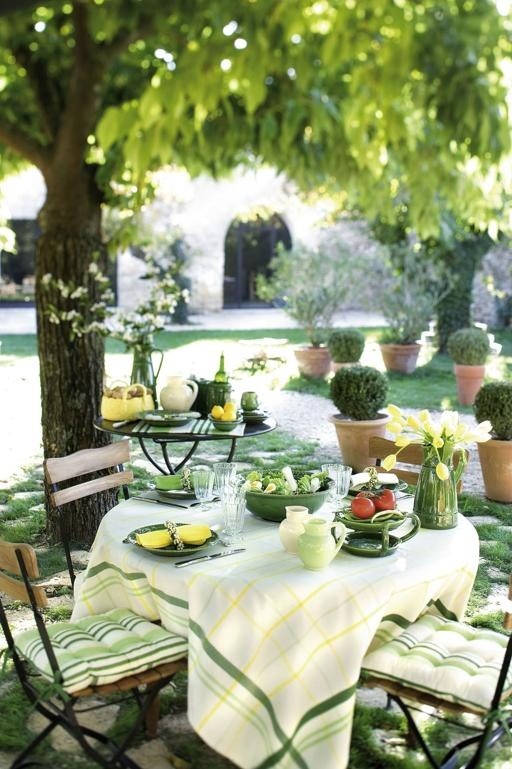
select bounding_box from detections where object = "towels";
[135,525,211,548]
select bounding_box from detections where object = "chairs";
[367,434,469,499]
[0,539,188,769]
[361,607,512,769]
[42,438,134,580]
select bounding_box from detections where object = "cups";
[241,391,262,411]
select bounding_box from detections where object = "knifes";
[175,549,249,569]
[132,495,189,510]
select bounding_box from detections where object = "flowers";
[380,401,480,481]
[38,243,191,343]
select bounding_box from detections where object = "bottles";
[214,352,226,383]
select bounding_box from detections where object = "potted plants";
[369,236,455,372]
[446,327,489,407]
[331,367,391,473]
[253,228,368,377]
[327,328,365,377]
[471,380,512,503]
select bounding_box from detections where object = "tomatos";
[350,488,395,519]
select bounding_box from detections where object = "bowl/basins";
[207,413,243,431]
[247,470,337,523]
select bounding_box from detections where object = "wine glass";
[193,462,248,547]
[321,464,352,511]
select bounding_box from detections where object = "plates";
[238,411,272,424]
[350,479,409,497]
[154,481,193,500]
[134,410,201,426]
[122,521,219,558]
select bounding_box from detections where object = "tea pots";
[279,505,347,571]
[160,373,198,412]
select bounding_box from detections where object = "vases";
[412,443,460,530]
[131,333,164,411]
